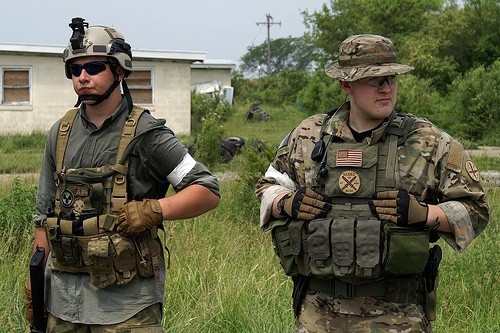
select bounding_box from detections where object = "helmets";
[61,17,133,79]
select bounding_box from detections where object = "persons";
[25,18,220,333]
[254,33,489,333]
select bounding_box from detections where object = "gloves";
[369,189,429,227]
[277,186,332,220]
[24,284,35,325]
[117,197,163,239]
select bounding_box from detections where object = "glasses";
[66,59,114,78]
[355,74,399,86]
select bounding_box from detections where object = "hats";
[325,33,415,81]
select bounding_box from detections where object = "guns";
[426,245,442,321]
[29,247,49,333]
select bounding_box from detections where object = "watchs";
[277,192,291,219]
